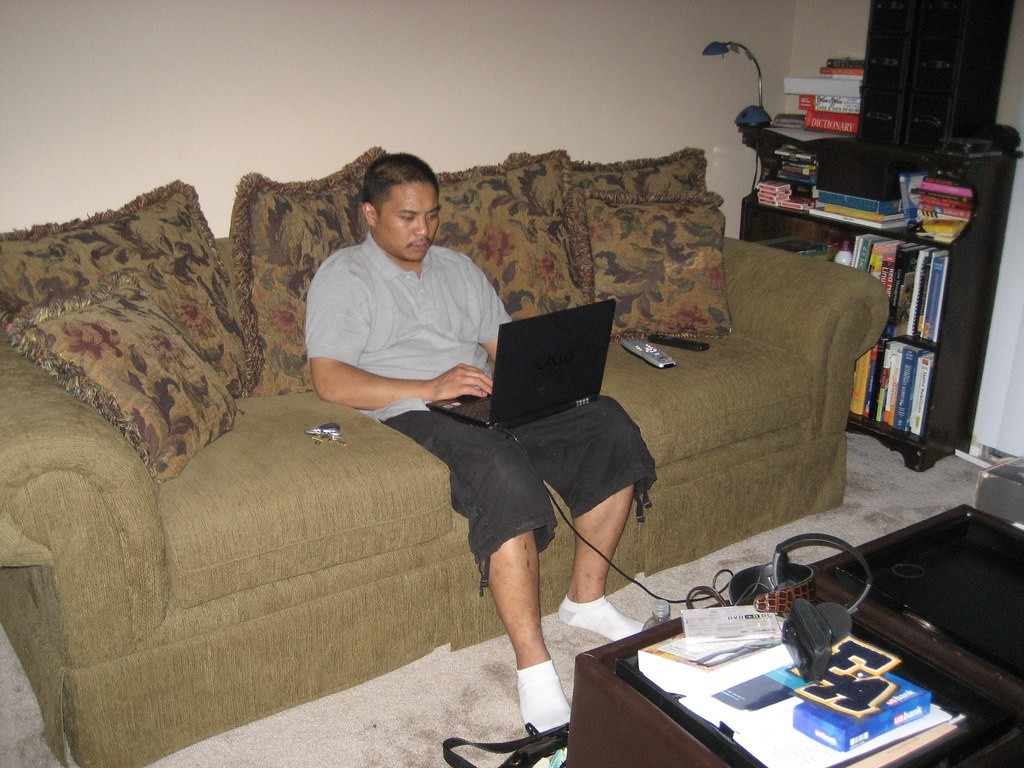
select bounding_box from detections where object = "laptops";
[425,299,616,430]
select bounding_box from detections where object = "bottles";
[642,599,671,632]
[834,241,853,267]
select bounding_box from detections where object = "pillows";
[233,147,386,396]
[431,150,588,321]
[572,147,711,194]
[0,178,232,299]
[6,272,238,484]
[583,188,734,339]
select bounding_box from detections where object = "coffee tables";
[546,504,1024,768]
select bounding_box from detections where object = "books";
[753,215,950,343]
[810,203,906,228]
[774,145,817,205]
[850,338,936,438]
[898,170,972,223]
[636,616,793,695]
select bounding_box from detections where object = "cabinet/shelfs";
[738,0,1021,474]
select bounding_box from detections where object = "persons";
[305,153,657,731]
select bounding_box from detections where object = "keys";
[305,422,347,446]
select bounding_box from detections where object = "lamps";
[703,40,772,128]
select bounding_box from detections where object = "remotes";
[647,334,710,352]
[621,339,677,368]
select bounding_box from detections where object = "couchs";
[0,238,891,768]
